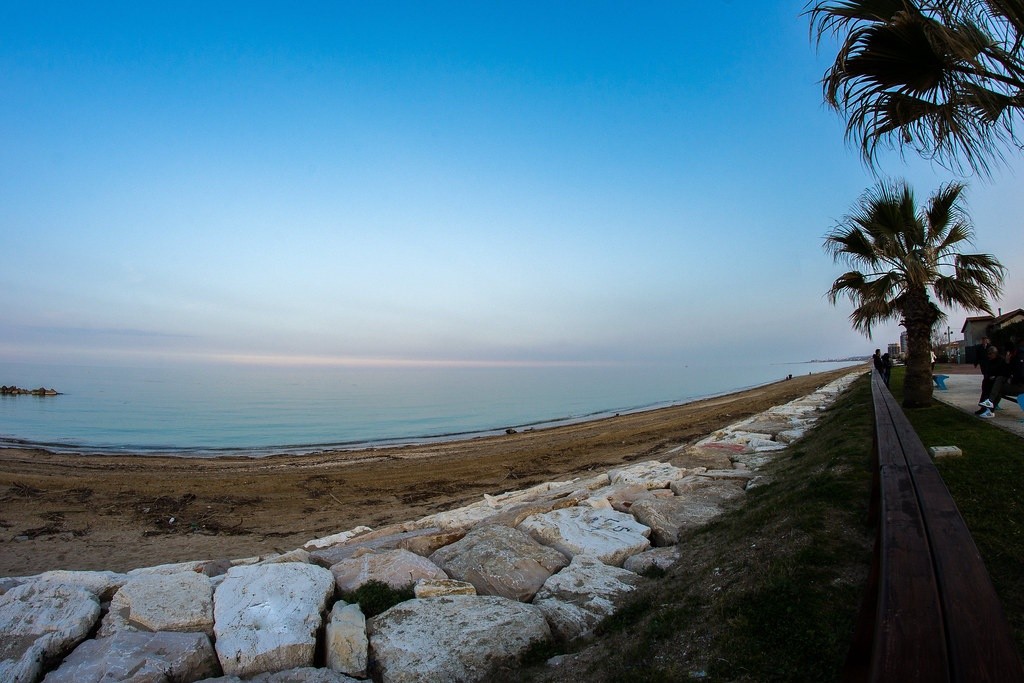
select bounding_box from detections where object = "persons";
[930,350,937,371]
[872,349,892,389]
[973,337,1024,419]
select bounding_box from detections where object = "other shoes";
[975,406,987,415]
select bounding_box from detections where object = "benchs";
[1002,393,1024,423]
[933,375,949,390]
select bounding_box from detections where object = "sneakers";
[978,398,994,409]
[979,408,995,419]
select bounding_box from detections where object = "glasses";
[987,352,991,355]
